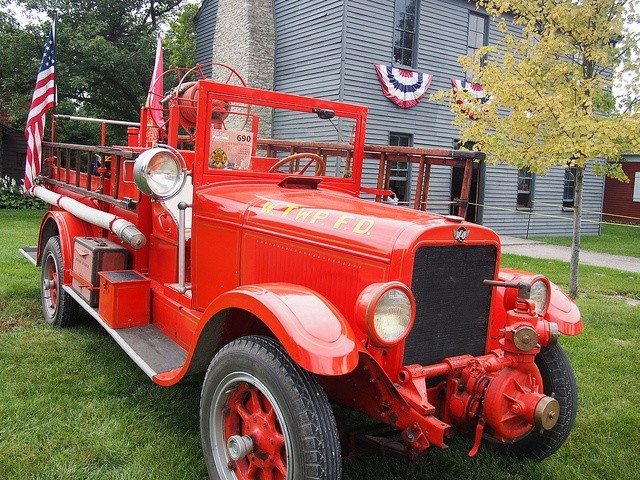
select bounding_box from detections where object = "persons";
[387,187,399,205]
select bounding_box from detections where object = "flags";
[24,21,58,197]
[138,38,166,145]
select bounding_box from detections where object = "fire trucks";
[18,59,584,480]
